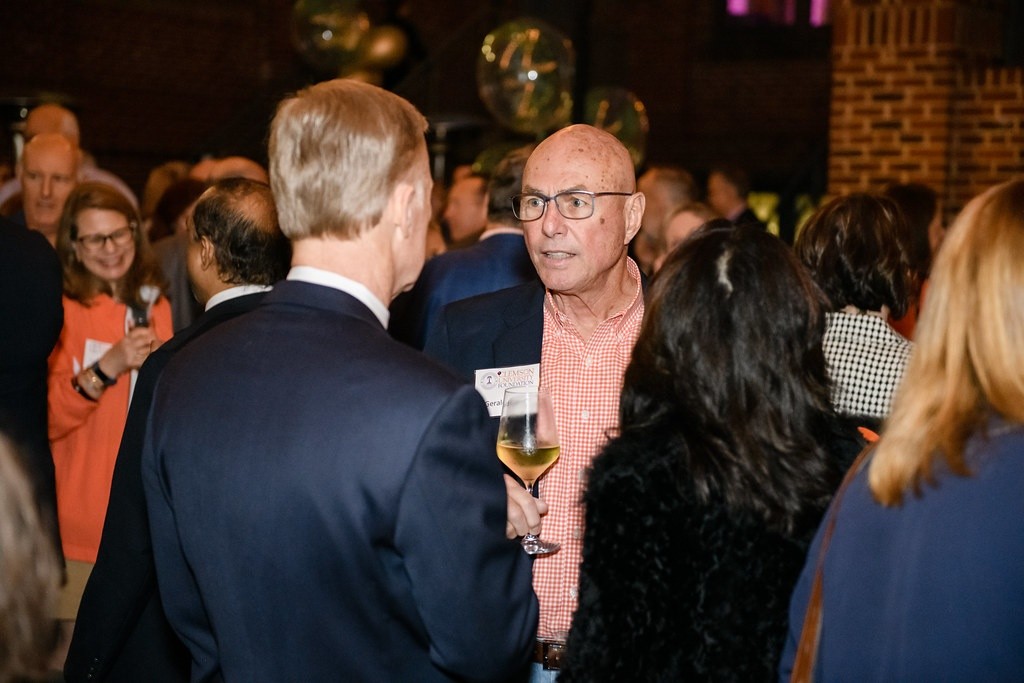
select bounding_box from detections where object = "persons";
[48,179,173,658]
[0,431,73,683]
[141,79,541,683]
[550,222,863,683]
[1,107,948,419]
[429,122,661,683]
[61,175,296,682]
[779,178,1024,683]
[0,133,80,588]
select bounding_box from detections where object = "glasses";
[72,222,134,252]
[508,191,632,222]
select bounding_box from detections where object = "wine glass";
[496,386,560,554]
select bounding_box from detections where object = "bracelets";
[84,361,117,392]
